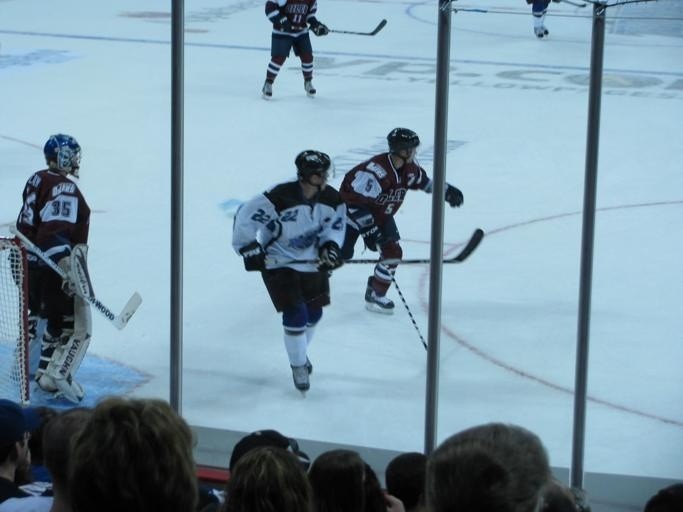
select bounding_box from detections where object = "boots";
[365,279,395,309]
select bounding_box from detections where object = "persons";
[1,398,591,510]
[527,0,560,39]
[233,149,348,392]
[261,0,329,95]
[9,132,95,404]
[339,127,463,308]
[645,483,683,512]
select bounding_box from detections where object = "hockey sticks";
[264,229,483,266]
[291,19,386,35]
[7,225,142,329]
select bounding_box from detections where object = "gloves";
[311,22,328,36]
[360,225,384,252]
[283,21,296,32]
[57,256,82,300]
[314,244,343,272]
[444,183,464,208]
[240,242,266,272]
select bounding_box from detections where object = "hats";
[0,397,42,453]
[228,428,311,485]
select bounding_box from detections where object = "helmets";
[294,149,331,176]
[387,127,421,151]
[42,133,81,179]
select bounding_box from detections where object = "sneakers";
[534,22,548,37]
[263,79,273,96]
[303,79,316,94]
[290,357,313,390]
